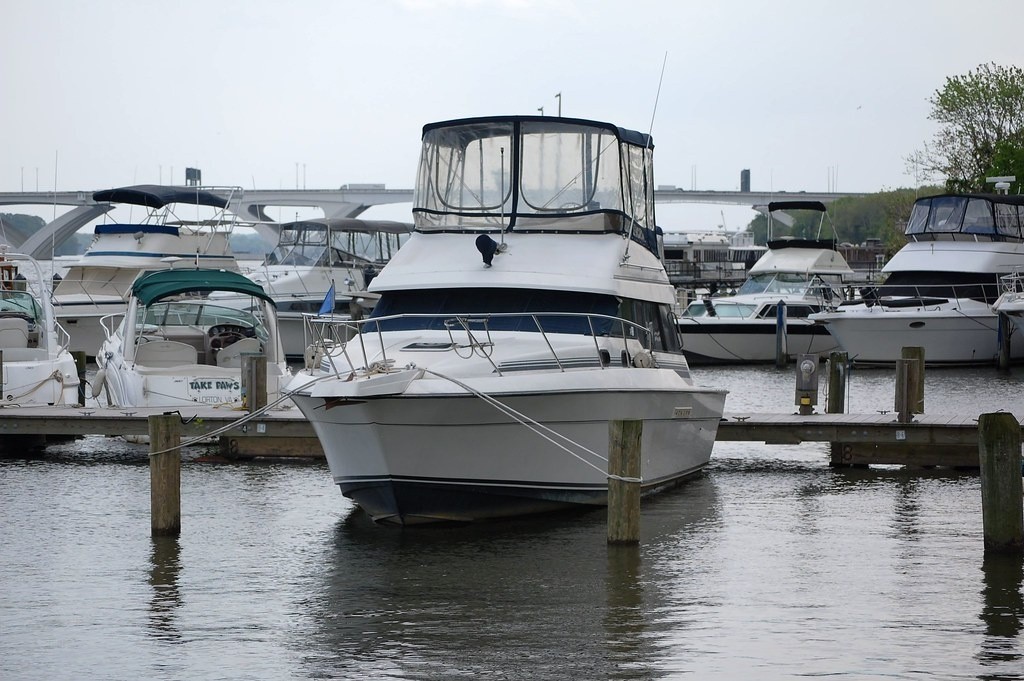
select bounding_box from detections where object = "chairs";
[134,341,197,367]
[0,318,29,348]
[216,338,260,368]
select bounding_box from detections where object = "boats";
[0,167,421,450]
[802,174,1024,368]
[668,196,861,368]
[283,112,733,522]
[992,268,1024,349]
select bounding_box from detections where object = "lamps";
[476,235,497,266]
[134,232,144,244]
